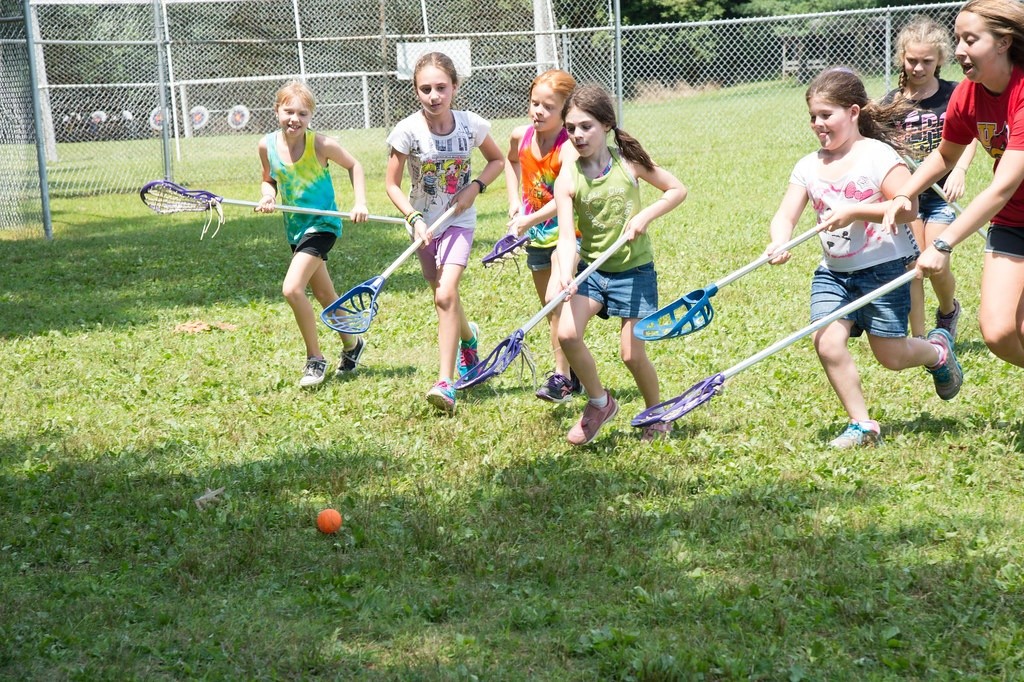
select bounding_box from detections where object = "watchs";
[932,239,953,252]
[472,179,486,194]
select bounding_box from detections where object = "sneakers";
[936,298,962,337]
[426,378,457,412]
[535,367,586,402]
[829,419,883,450]
[567,389,617,445]
[300,355,329,387]
[924,329,963,401]
[642,420,673,442]
[335,334,366,374]
[455,322,481,382]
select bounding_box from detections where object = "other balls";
[316,509,340,533]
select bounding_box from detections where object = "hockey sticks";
[482,233,532,264]
[631,269,919,427]
[450,229,630,392]
[138,178,406,225]
[319,201,459,335]
[900,151,988,240]
[632,191,887,341]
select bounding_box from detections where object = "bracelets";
[407,210,424,226]
[893,195,911,201]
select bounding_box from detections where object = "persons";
[555,82,687,447]
[252,80,367,387]
[864,14,978,371]
[878,0,1024,367]
[505,67,584,402]
[383,51,506,413]
[764,69,964,450]
